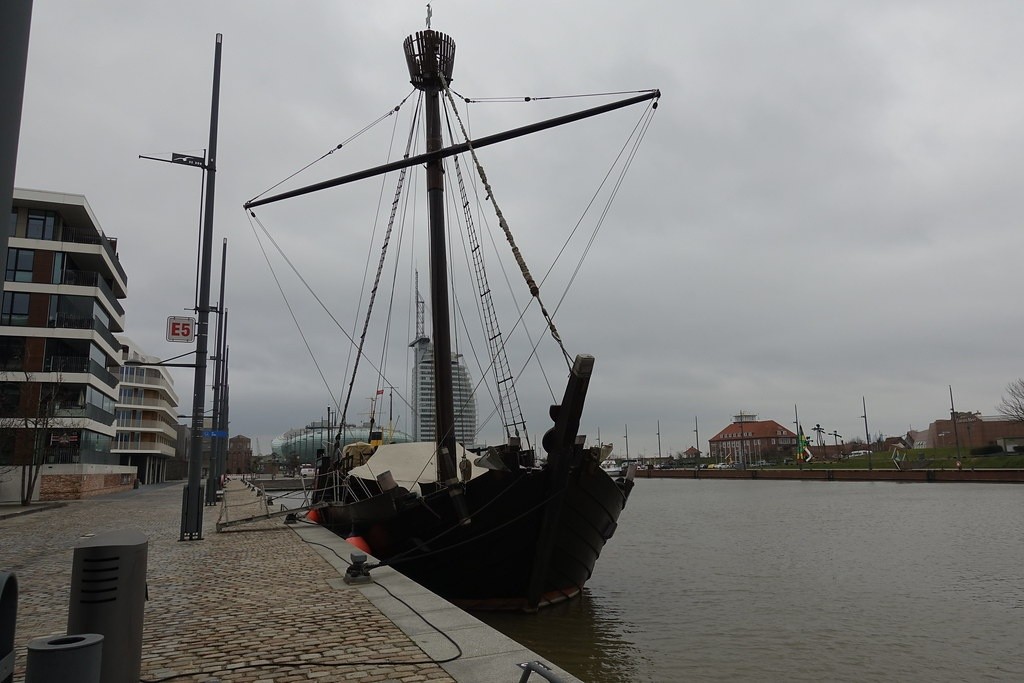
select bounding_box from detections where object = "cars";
[277,460,318,480]
[624,450,875,472]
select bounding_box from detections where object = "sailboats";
[241,4,664,608]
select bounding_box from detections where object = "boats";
[600,458,623,477]
